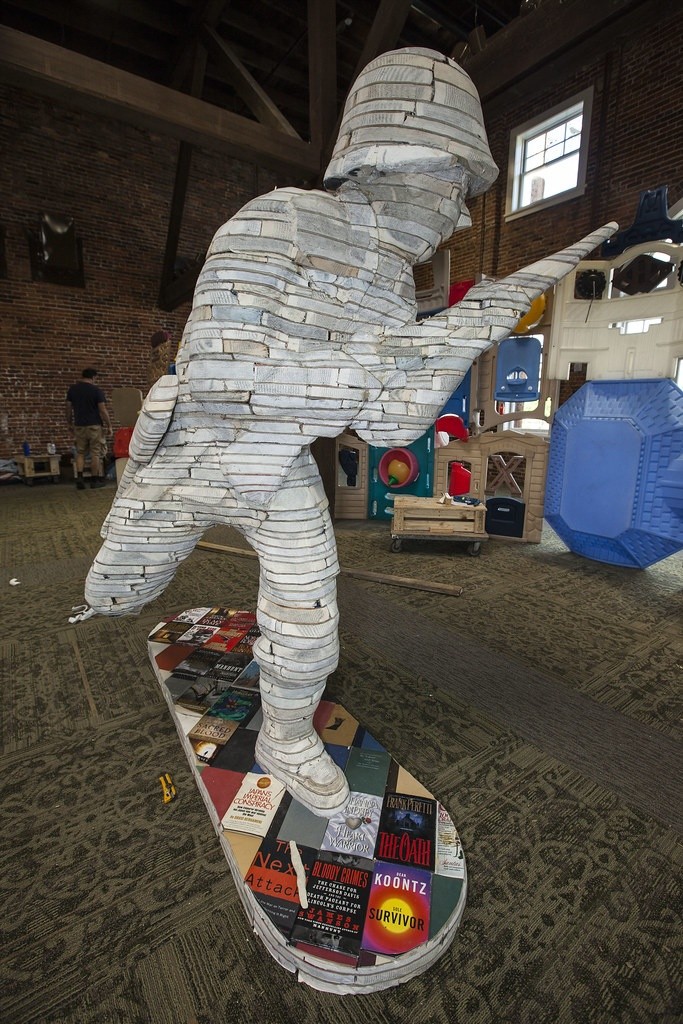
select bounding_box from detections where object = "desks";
[13,453,62,485]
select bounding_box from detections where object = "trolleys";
[390,435,489,558]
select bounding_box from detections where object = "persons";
[82,46,622,816]
[66,367,113,490]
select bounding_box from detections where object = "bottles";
[23,440,30,456]
[47,441,55,455]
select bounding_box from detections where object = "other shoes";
[90,479,105,488]
[77,478,85,489]
